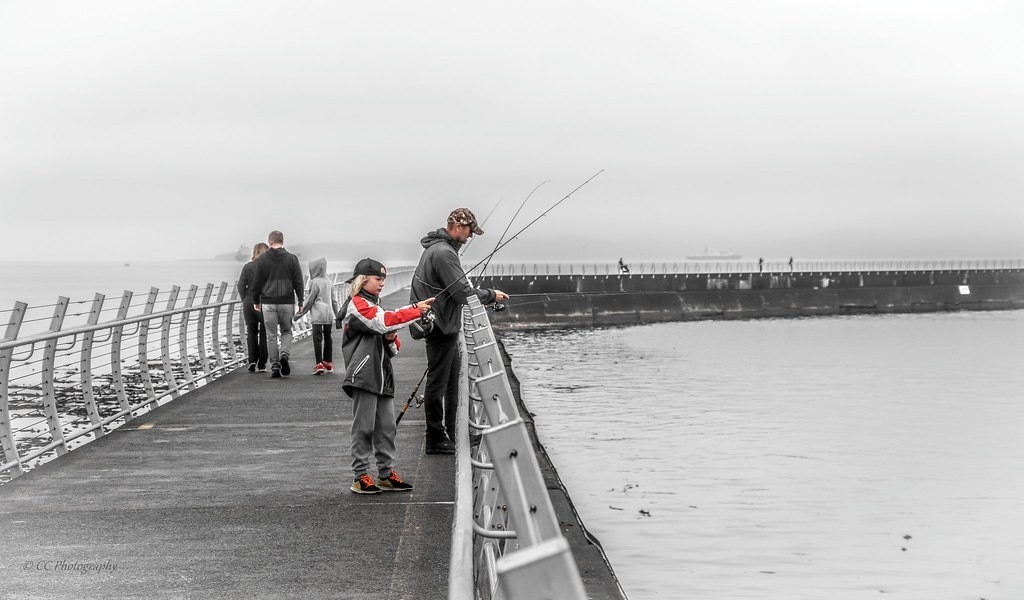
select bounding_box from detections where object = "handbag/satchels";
[409,318,435,339]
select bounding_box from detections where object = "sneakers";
[376,470,414,491]
[450,432,482,447]
[426,438,455,454]
[350,474,383,494]
[313,363,324,375]
[323,361,333,373]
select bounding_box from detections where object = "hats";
[344,258,386,283]
[447,208,484,235]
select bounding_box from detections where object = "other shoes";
[280,354,290,375]
[271,366,281,378]
[259,365,266,372]
[248,362,256,371]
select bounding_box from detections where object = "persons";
[336,258,436,495]
[293,258,339,375]
[788,257,794,272]
[238,231,305,377]
[759,257,765,272]
[408,208,510,455]
[619,258,625,271]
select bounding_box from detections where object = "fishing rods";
[457,198,504,258]
[428,167,607,296]
[390,178,550,432]
